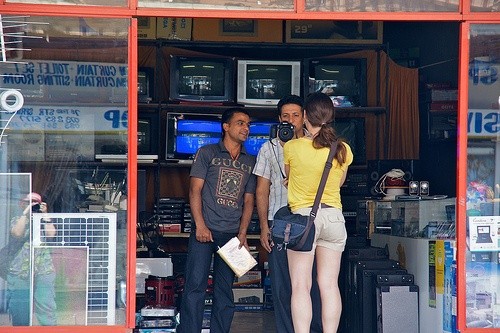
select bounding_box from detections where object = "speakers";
[366,158,414,198]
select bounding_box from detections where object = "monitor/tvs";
[220,19,257,36]
[303,57,368,108]
[242,119,280,156]
[329,117,367,165]
[93,107,161,163]
[168,55,235,104]
[237,60,301,108]
[110,66,154,103]
[165,112,224,165]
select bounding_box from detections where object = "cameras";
[270,121,294,142]
[32,204,40,211]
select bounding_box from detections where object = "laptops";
[476,226,493,243]
